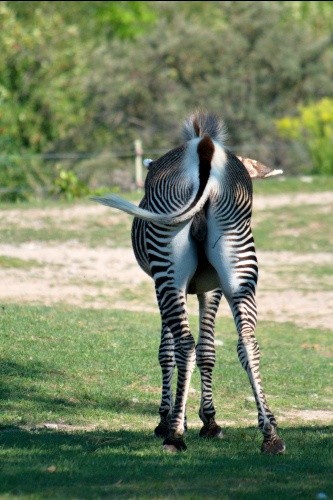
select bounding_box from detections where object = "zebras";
[88,109,289,456]
